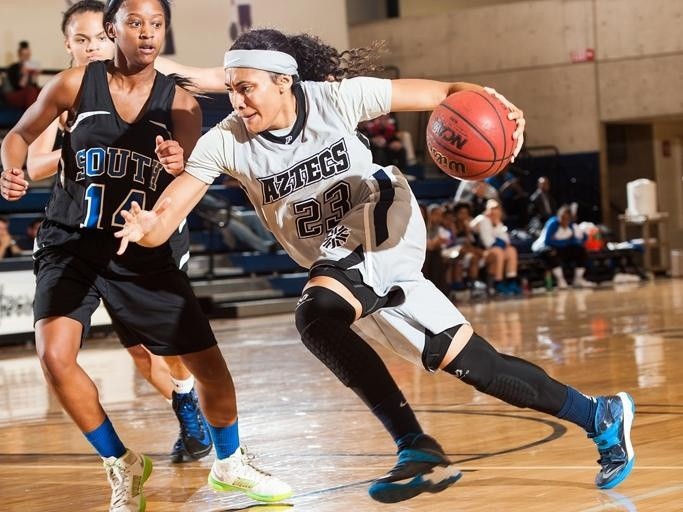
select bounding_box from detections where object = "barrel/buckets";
[626,176,657,217]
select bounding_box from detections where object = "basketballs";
[425,88,516,181]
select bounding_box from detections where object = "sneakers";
[558,278,567,289]
[450,278,521,301]
[587,392,635,489]
[368,434,462,503]
[170,387,213,463]
[104,447,152,512]
[208,441,292,501]
[571,277,597,288]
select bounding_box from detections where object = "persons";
[0,220,21,258]
[196,193,279,259]
[113,26,637,504]
[23,214,42,250]
[365,115,409,173]
[26,0,336,463]
[423,170,611,301]
[7,37,37,89]
[0,0,293,512]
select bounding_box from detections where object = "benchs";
[186,84,310,297]
[0,101,54,268]
[405,169,639,300]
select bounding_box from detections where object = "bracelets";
[7,240,17,247]
